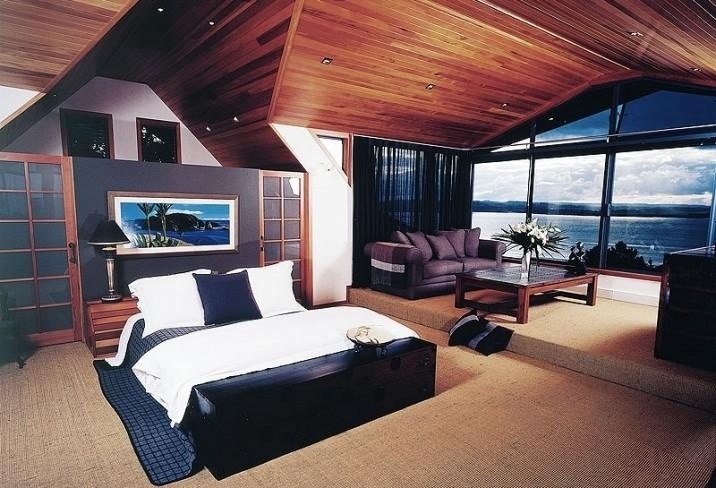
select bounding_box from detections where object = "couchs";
[372,227,507,301]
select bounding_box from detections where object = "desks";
[455,265,600,324]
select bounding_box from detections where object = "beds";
[92,260,437,486]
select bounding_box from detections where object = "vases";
[521,251,530,278]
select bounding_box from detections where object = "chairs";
[0,292,23,369]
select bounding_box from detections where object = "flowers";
[491,216,570,268]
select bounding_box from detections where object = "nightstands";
[81,297,140,358]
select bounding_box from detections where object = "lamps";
[87,221,130,302]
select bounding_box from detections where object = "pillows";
[192,270,262,326]
[128,269,212,339]
[461,317,513,354]
[392,227,481,262]
[448,308,478,343]
[225,260,308,316]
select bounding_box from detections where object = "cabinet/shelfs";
[654,245,715,372]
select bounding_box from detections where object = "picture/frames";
[106,191,239,259]
